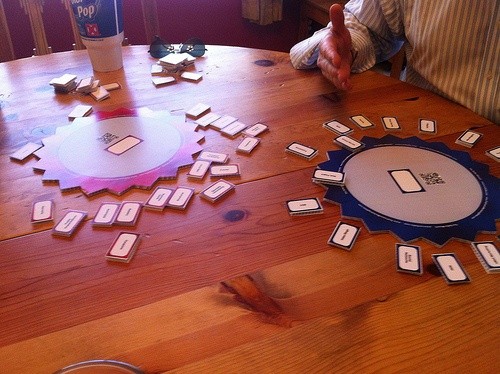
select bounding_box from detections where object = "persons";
[290,0,500,126]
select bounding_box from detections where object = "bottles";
[71,0,124,73]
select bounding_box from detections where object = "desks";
[0,44,500,373]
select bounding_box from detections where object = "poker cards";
[14,49,268,267]
[285,106,500,289]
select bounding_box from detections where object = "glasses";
[147,35,208,58]
